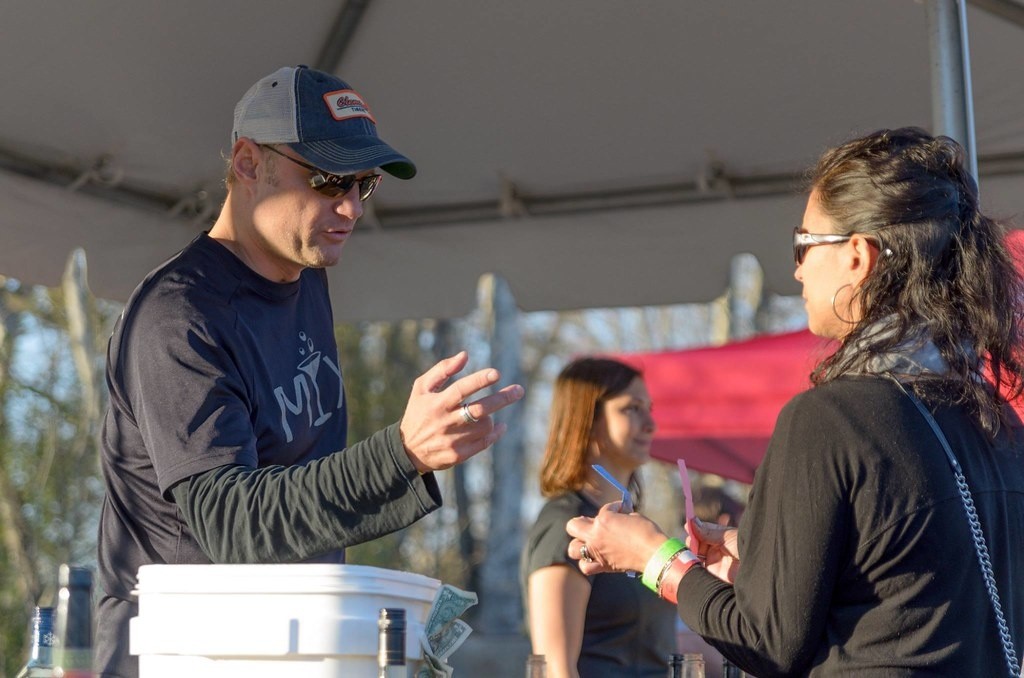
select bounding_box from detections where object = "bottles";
[667,654,705,678]
[525,654,546,678]
[722,657,746,678]
[16,607,52,677]
[377,608,408,678]
[50,563,97,678]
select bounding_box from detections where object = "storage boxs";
[127,561,446,678]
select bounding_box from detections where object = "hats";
[229,65,418,180]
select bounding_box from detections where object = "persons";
[562,125,1022,678]
[520,358,680,678]
[90,62,527,678]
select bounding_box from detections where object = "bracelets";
[641,538,687,593]
[657,550,702,604]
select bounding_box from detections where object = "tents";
[591,226,1023,485]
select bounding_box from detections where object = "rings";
[463,403,480,424]
[581,545,592,563]
[697,554,707,559]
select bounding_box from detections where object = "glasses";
[257,144,382,202]
[792,225,880,266]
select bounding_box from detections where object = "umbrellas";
[0,2,1024,323]
